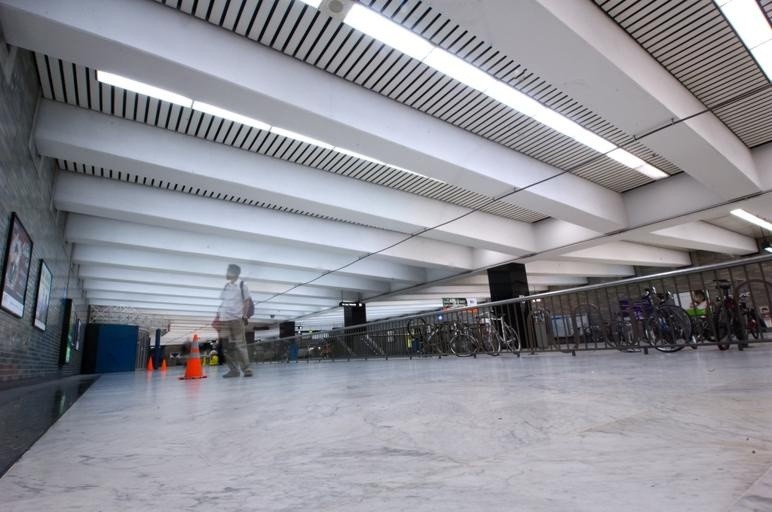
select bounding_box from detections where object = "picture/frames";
[1,212,54,333]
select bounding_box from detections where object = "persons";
[688,289,706,307]
[212,263,254,378]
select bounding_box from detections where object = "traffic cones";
[160,359,167,371]
[147,355,154,371]
[177,332,207,380]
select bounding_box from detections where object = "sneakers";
[244,369,252,376]
[222,371,241,377]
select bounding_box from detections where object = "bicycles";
[406,303,524,359]
[572,268,765,352]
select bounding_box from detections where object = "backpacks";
[223,280,255,319]
